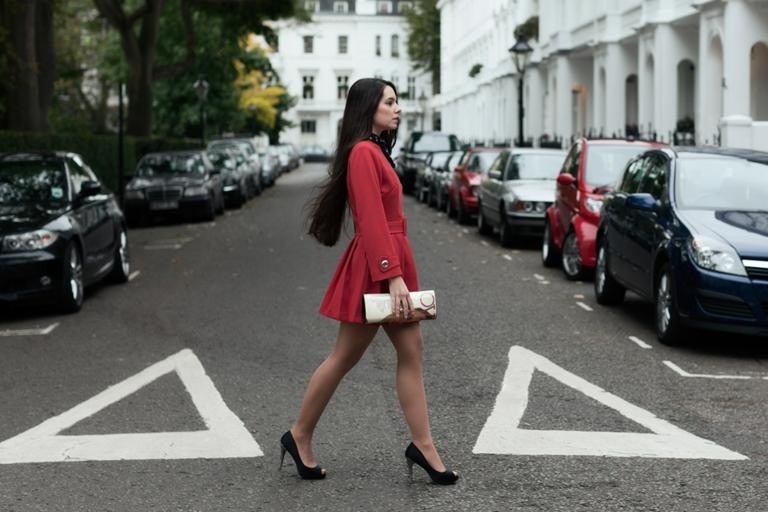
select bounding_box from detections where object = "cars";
[1,147,131,320]
[126,137,335,230]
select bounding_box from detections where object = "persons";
[278,76,460,486]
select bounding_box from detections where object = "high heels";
[402,441,459,484]
[275,427,328,482]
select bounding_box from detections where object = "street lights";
[248,100,256,138]
[508,35,538,148]
[192,75,208,146]
[417,88,431,135]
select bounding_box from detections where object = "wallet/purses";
[361,288,439,328]
